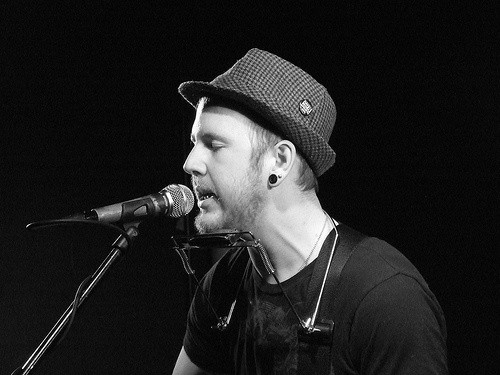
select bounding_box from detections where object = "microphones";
[81,183,195,224]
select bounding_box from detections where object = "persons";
[171,48,449,375]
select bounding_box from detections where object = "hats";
[178,48,337,178]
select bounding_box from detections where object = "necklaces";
[298,214,328,273]
